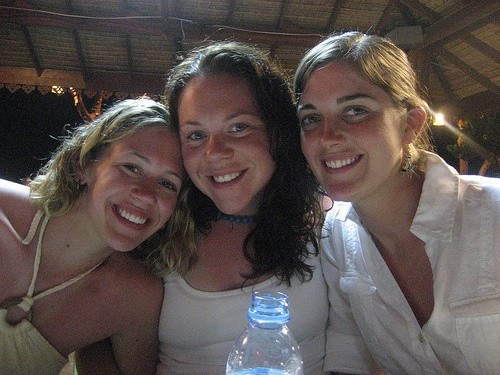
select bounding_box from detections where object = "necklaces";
[218,213,257,225]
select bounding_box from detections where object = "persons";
[74,42,330,375]
[0,98,195,375]
[294,31,500,374]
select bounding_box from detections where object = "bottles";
[225,290,304,375]
